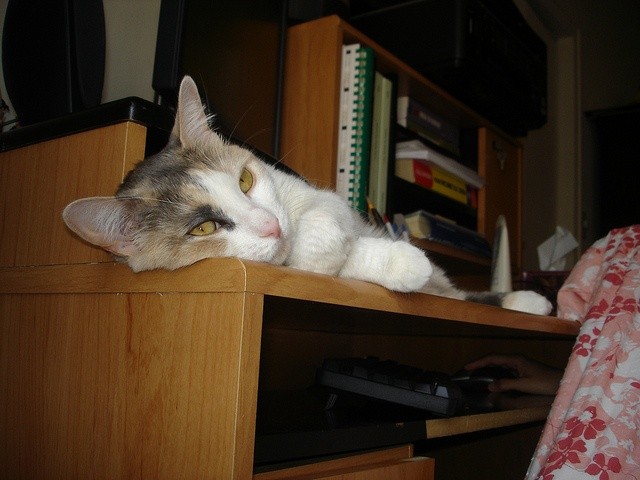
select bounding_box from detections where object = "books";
[339,41,491,276]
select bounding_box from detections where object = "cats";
[60,73,556,316]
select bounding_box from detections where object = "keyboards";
[318,354,511,413]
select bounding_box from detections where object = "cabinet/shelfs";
[279,12,523,277]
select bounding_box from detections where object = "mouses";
[450,363,525,399]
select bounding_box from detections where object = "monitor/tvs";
[150,1,287,167]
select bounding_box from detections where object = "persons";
[456,354,565,396]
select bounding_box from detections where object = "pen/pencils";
[362,197,410,241]
